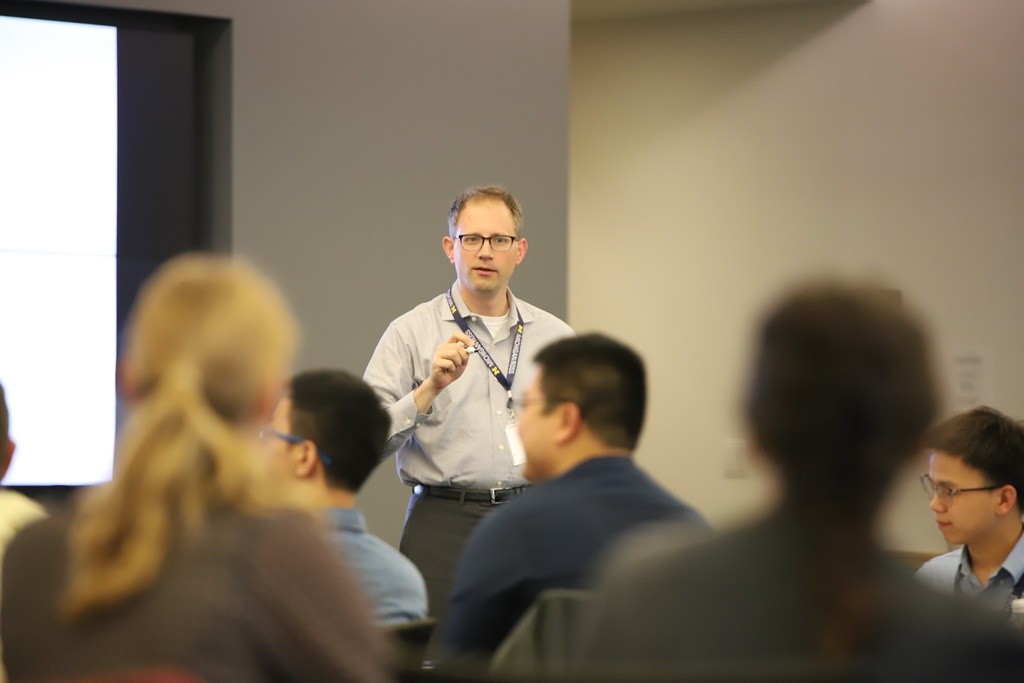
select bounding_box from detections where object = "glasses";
[454,233,521,253]
[917,476,1005,504]
[253,425,336,468]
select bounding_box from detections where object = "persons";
[4,248,397,683]
[583,271,1024,683]
[362,185,585,681]
[0,378,49,570]
[246,368,429,635]
[421,332,717,683]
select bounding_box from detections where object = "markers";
[463,344,480,355]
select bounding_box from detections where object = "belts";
[411,481,534,505]
